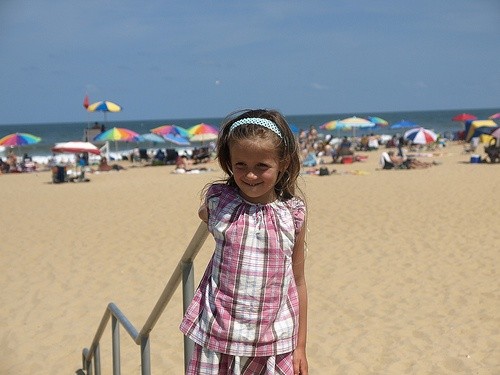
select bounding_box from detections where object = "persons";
[179,108,310,375]
[129,146,210,169]
[296,124,317,153]
[76,155,85,180]
[320,133,419,166]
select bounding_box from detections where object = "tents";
[465,119,498,144]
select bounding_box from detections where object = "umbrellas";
[450,113,478,121]
[93,122,220,143]
[388,120,420,129]
[0,131,42,148]
[52,141,101,156]
[319,115,389,130]
[87,100,123,113]
[489,114,500,120]
[403,127,438,145]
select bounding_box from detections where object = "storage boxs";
[342,157,352,164]
[471,154,482,163]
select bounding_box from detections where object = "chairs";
[53,166,68,183]
